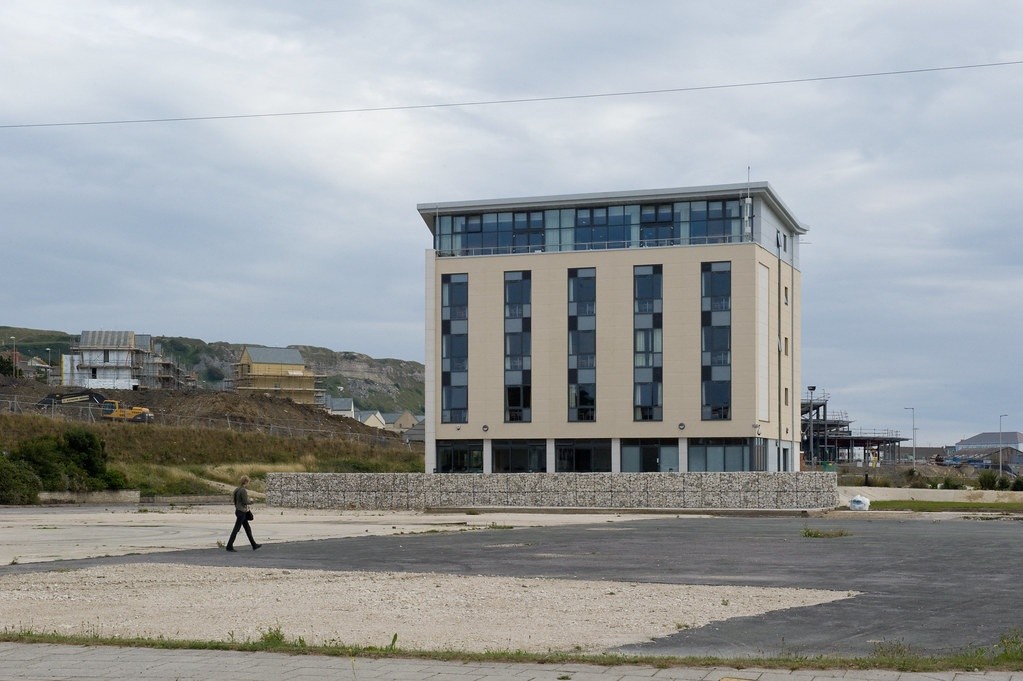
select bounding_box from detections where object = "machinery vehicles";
[34,388,155,424]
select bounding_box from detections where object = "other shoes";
[253,544,262,550]
[226,548,237,552]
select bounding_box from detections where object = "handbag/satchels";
[245,510,254,520]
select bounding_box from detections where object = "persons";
[226,475,263,552]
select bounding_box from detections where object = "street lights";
[46,348,51,385]
[807,386,817,471]
[9,336,16,378]
[1000,415,1009,477]
[904,407,916,471]
